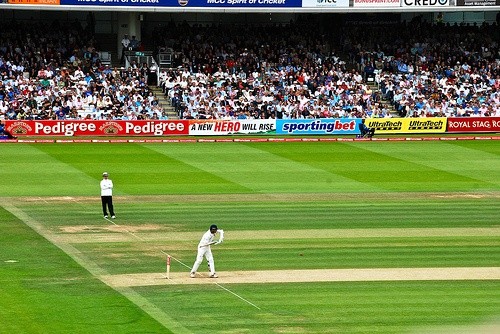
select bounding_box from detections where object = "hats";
[102,172,108,176]
[210,224,217,231]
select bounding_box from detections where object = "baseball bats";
[199,240,218,248]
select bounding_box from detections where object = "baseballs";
[300,253,303,256]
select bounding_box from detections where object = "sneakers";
[209,273,218,278]
[111,215,116,219]
[104,215,109,218]
[190,271,196,278]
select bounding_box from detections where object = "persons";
[100,172,116,219]
[189,224,219,278]
[0,12,500,120]
